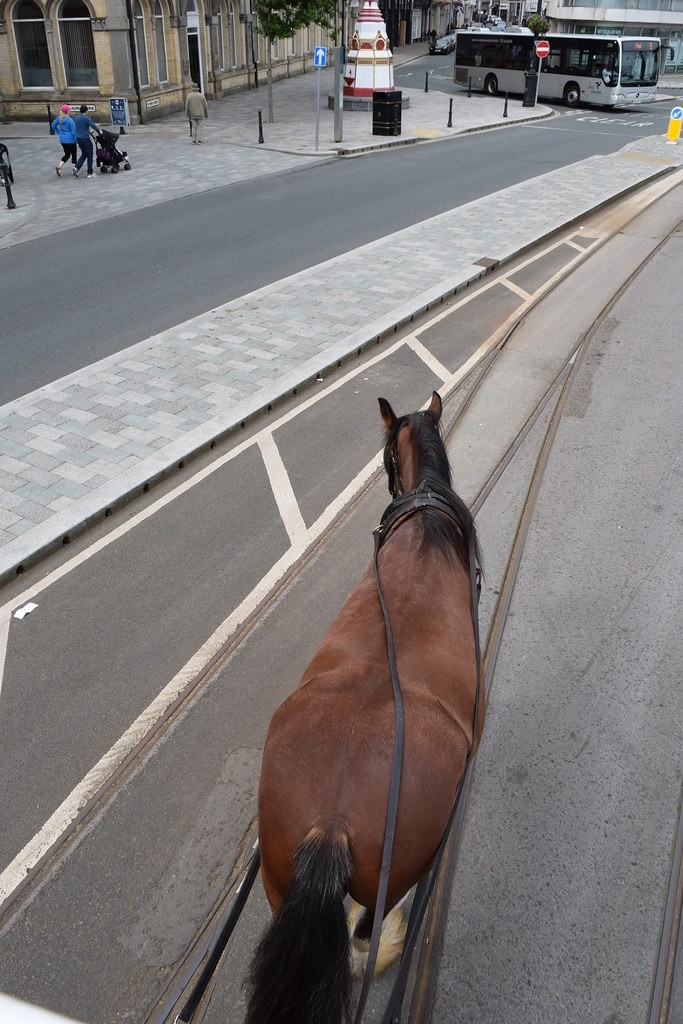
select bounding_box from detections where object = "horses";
[244,391,488,1024]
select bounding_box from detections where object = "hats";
[80,104,88,113]
[61,105,72,111]
[190,83,200,90]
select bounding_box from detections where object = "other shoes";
[193,141,196,145]
[56,166,61,176]
[198,141,201,144]
[73,172,81,175]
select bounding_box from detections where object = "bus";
[453,31,675,110]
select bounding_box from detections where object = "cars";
[453,14,534,37]
[443,35,457,51]
[429,38,452,55]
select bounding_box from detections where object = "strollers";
[89,129,132,174]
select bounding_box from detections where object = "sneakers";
[72,167,79,177]
[87,172,96,178]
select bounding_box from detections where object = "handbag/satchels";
[96,148,110,159]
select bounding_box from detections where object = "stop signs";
[536,41,551,58]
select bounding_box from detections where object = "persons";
[432,28,437,43]
[185,82,209,145]
[475,50,482,66]
[73,105,103,178]
[51,105,77,178]
[603,62,619,83]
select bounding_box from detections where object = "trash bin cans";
[371,90,403,137]
[523,69,538,107]
